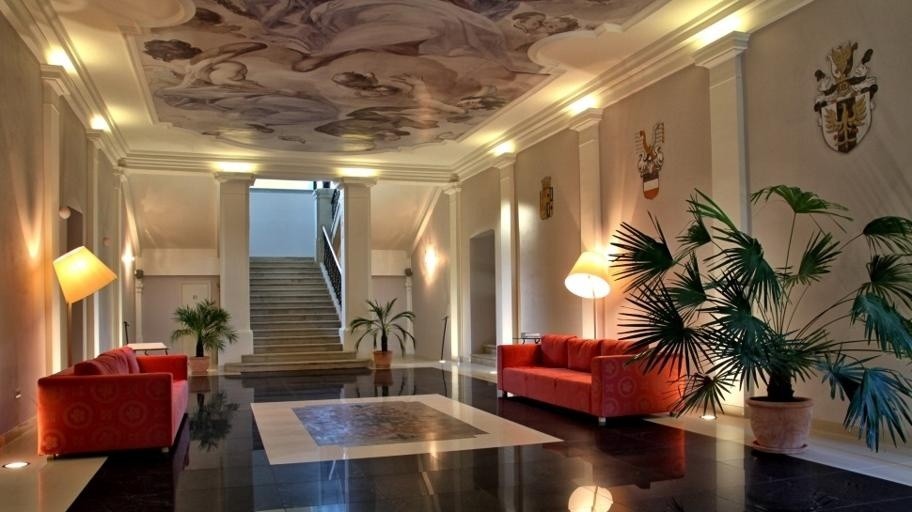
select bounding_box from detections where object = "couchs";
[36,346,189,461]
[494,396,688,488]
[496,333,685,428]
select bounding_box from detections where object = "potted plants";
[169,299,238,372]
[356,370,416,396]
[608,182,912,454]
[190,390,239,454]
[349,296,416,366]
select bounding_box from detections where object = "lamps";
[53,245,119,361]
[564,251,616,339]
[566,485,616,512]
